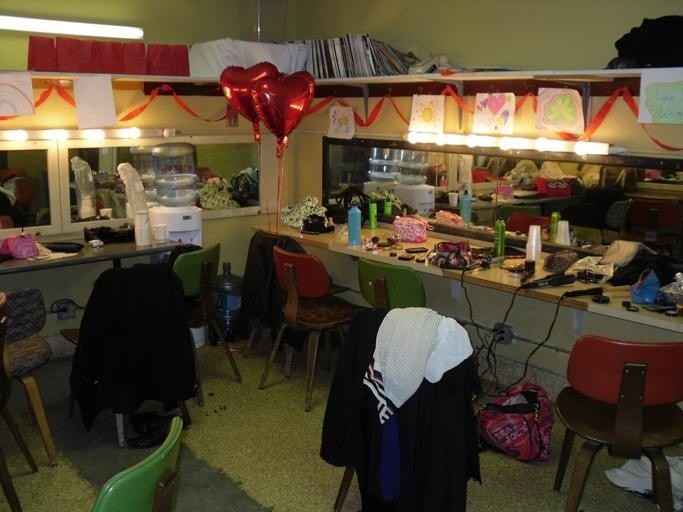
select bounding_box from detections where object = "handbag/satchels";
[393,216,427,243]
[631,269,660,304]
[281,197,334,235]
[198,173,258,209]
[436,210,462,225]
[0,235,39,259]
[427,241,472,269]
[476,383,553,461]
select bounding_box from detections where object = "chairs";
[59,263,197,448]
[497,197,682,246]
[332,303,469,510]
[355,255,426,314]
[3,315,39,510]
[2,288,58,466]
[240,230,351,379]
[256,242,377,412]
[552,339,682,510]
[173,241,242,407]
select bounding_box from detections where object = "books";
[272,32,436,77]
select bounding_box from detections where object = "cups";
[100,208,112,219]
[524,223,543,277]
[153,223,167,243]
[81,196,94,219]
[448,192,458,207]
[135,210,151,246]
[555,220,570,245]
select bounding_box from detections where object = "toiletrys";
[494,216,506,257]
[551,211,561,244]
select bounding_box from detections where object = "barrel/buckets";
[151,141,199,206]
[210,261,245,347]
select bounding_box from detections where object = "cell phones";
[406,247,427,254]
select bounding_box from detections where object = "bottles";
[348,200,362,246]
[459,190,472,226]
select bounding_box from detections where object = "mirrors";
[321,135,683,274]
[0,132,262,241]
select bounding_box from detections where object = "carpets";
[13,398,272,511]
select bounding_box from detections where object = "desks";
[253,224,333,250]
[0,236,192,275]
[260,224,683,334]
[471,194,560,211]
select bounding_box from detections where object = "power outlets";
[494,323,510,344]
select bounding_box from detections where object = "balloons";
[249,70,315,143]
[219,61,280,123]
